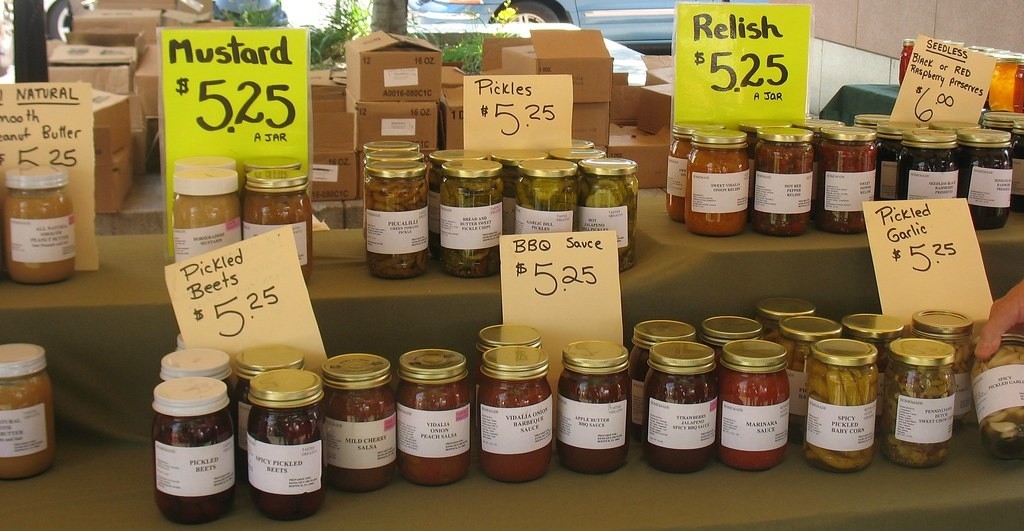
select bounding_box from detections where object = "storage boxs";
[41,0,674,215]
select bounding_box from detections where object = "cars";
[403,0,771,53]
[41,0,293,39]
